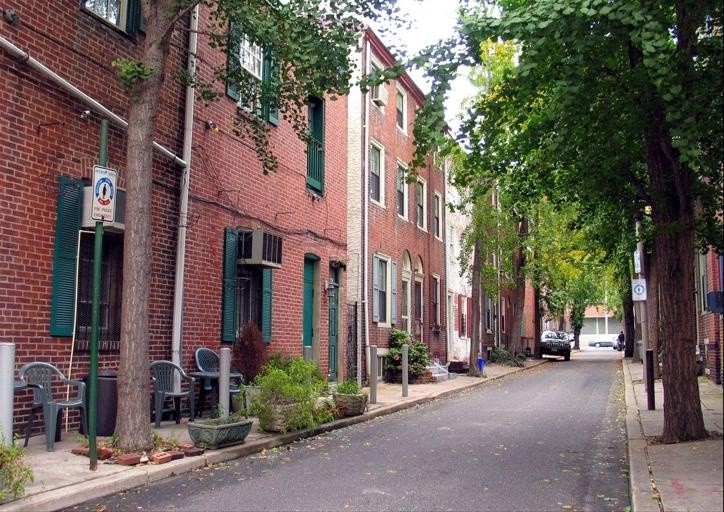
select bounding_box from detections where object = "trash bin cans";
[81,372,117,436]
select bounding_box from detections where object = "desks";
[14,381,26,390]
[188,371,242,418]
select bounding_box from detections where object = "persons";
[618,330,624,349]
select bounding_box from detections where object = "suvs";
[539,328,572,361]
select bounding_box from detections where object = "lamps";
[327,283,334,297]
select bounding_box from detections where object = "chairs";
[195,347,243,418]
[150,360,195,428]
[18,361,89,452]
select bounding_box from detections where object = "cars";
[588,340,614,348]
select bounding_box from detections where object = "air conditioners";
[372,86,388,106]
[81,185,126,231]
[236,229,283,268]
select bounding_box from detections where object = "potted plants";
[384,328,434,385]
[235,353,328,435]
[0,436,34,504]
[187,409,253,451]
[334,380,368,416]
